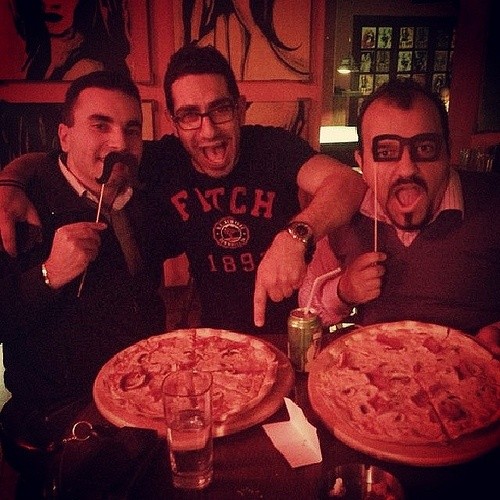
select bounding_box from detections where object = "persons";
[0,71,185,500]
[296,78,500,359]
[0,45,368,336]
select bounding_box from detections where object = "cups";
[314,464,404,500]
[160,370,214,490]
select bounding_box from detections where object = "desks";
[50,331,500,500]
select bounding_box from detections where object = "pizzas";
[102,328,280,422]
[315,321,500,440]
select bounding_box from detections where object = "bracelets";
[42,262,53,289]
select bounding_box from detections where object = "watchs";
[278,222,315,251]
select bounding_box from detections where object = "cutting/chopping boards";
[93,326,296,441]
[305,318,500,467]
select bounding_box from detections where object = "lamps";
[336,37,354,74]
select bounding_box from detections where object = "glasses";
[173,97,240,130]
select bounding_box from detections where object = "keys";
[63,420,119,444]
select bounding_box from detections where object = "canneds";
[286,307,321,374]
